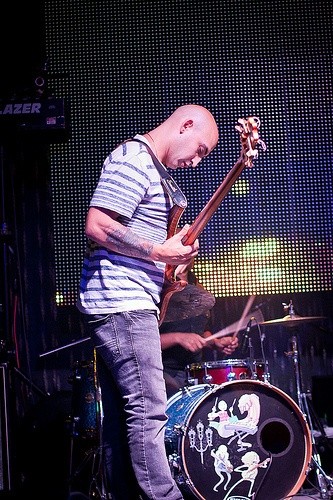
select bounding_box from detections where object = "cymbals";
[259,315,327,325]
[163,283,216,323]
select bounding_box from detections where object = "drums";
[186,359,267,385]
[164,380,312,500]
[70,359,105,444]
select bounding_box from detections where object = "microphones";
[241,320,251,353]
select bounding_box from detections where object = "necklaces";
[147,133,158,159]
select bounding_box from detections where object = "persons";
[156,265,238,400]
[77,103,219,500]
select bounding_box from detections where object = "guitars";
[159,115,267,328]
